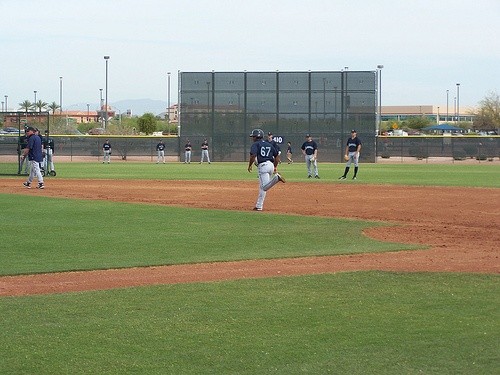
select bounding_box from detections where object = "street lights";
[103,55,110,129]
[59,76,64,112]
[446,89,449,122]
[377,64,385,136]
[5,95,8,112]
[34,90,37,112]
[456,83,460,115]
[100,98,104,128]
[167,72,172,123]
[99,88,103,99]
[1,102,4,112]
[87,104,90,122]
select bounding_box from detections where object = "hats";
[306,134,311,137]
[188,140,190,143]
[268,132,272,135]
[45,130,49,134]
[204,138,207,141]
[27,127,34,131]
[350,129,356,133]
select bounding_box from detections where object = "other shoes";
[338,176,347,180]
[308,175,312,179]
[277,174,286,183]
[351,177,356,179]
[314,175,320,179]
[255,207,262,211]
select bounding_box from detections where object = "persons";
[102,140,111,164]
[16,127,55,175]
[339,130,362,179]
[156,140,165,164]
[184,140,192,164]
[286,141,293,164]
[200,138,211,164]
[301,134,320,179]
[20,127,45,189]
[265,131,281,173]
[248,129,286,211]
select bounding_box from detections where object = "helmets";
[249,129,263,138]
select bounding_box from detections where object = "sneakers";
[36,183,44,189]
[23,181,32,188]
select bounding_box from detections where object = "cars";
[387,128,408,137]
